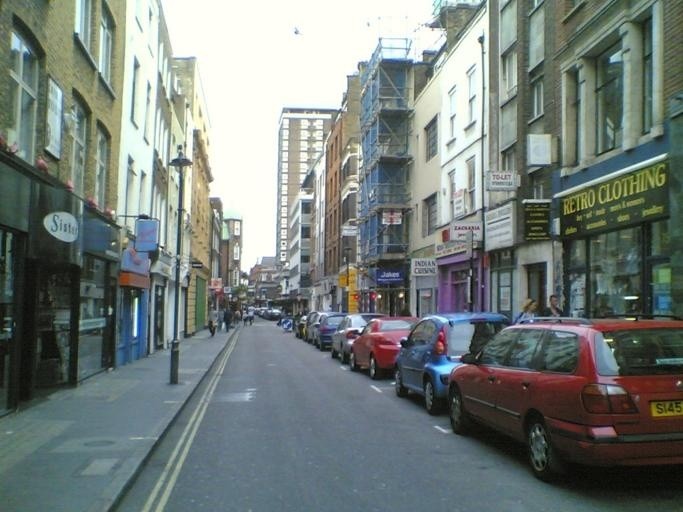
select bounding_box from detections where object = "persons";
[512,298,541,324]
[233,305,255,327]
[223,308,234,331]
[542,295,564,323]
[207,305,219,336]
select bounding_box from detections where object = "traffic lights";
[354,293,361,302]
[370,289,382,301]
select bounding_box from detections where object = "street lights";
[296,292,303,316]
[343,247,352,313]
[169,144,193,384]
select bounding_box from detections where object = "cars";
[248,306,282,320]
